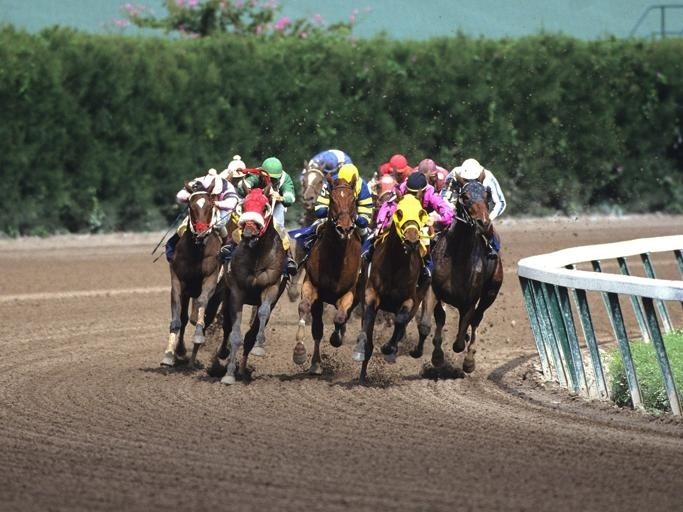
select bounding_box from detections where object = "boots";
[216,246,230,260]
[285,249,297,276]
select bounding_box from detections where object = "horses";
[159,176,226,369]
[286,158,329,304]
[290,173,363,377]
[349,187,431,381]
[372,165,398,206]
[422,167,441,192]
[226,170,235,183]
[213,177,291,386]
[407,168,505,373]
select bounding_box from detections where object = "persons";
[295,150,506,278]
[166,155,297,276]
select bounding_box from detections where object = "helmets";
[318,153,359,184]
[389,155,483,193]
[203,156,283,194]
